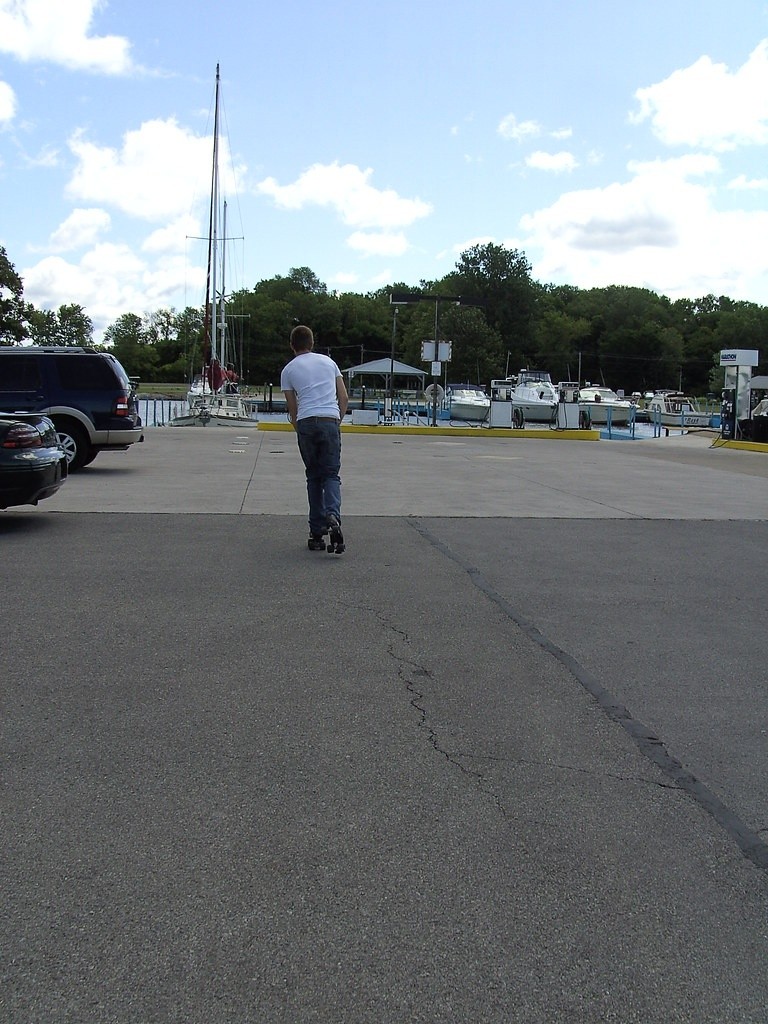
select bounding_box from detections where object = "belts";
[301,416,341,424]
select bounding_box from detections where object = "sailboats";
[170,59,262,429]
[428,347,724,432]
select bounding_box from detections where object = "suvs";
[0,346,145,472]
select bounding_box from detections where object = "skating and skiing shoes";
[322,514,346,554]
[307,532,326,551]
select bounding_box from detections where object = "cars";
[1,407,69,508]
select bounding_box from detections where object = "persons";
[281,326,348,554]
